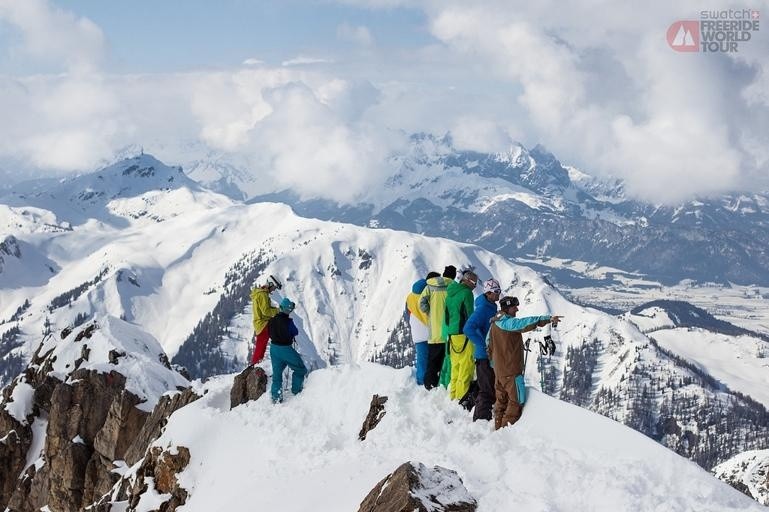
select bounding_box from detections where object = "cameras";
[552,322,557,328]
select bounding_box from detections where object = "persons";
[405,272,441,386]
[249,274,283,367]
[268,297,307,404]
[485,296,565,431]
[458,278,501,422]
[417,265,457,391]
[442,271,479,403]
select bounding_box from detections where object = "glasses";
[490,289,501,294]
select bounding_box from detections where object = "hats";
[267,271,285,290]
[279,297,295,310]
[443,265,457,280]
[482,278,501,290]
[498,295,520,307]
